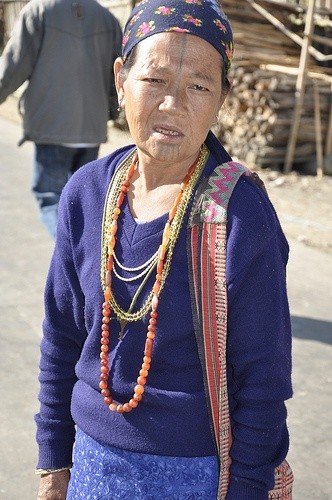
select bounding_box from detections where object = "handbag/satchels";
[216,457,294,500]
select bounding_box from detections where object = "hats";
[121,0,234,69]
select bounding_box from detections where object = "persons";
[0,0,123,241]
[35,1,295,500]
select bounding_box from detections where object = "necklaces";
[100,144,210,412]
